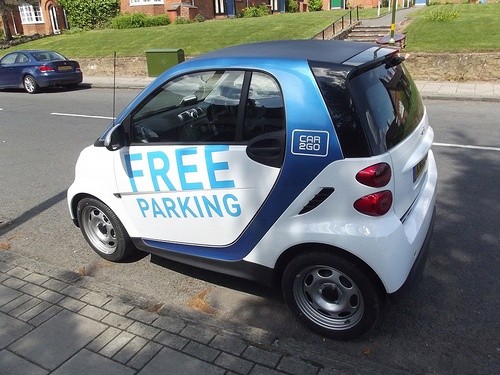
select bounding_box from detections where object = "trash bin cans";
[145,49,185,78]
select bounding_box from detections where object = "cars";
[0,49,84,94]
[66,38,441,342]
